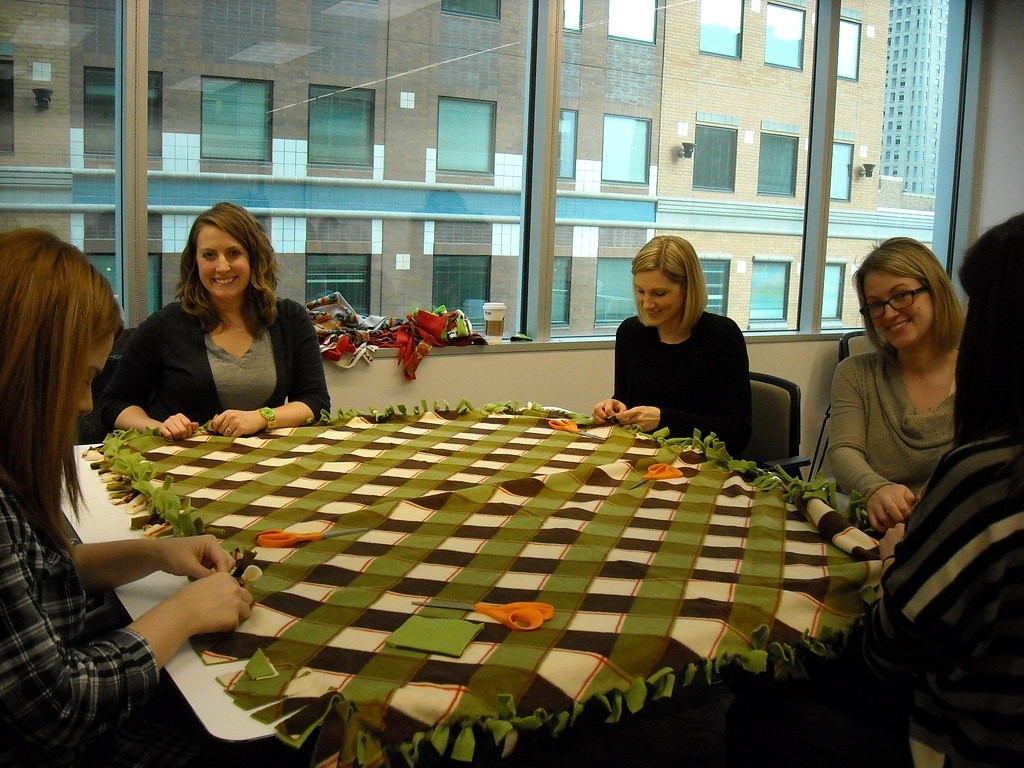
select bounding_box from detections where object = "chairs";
[742,372,811,481]
[808,330,876,484]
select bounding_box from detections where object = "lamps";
[859,163,876,177]
[678,142,696,159]
[32,88,54,111]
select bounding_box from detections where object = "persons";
[827,237,965,534]
[879,212,1024,768]
[591,235,754,459]
[96,201,331,441]
[0,226,255,768]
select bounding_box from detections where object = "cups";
[463,299,485,332]
[482,302,507,341]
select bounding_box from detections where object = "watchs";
[257,407,277,430]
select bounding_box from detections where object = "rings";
[226,428,233,434]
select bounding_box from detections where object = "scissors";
[548,419,605,441]
[411,600,556,631]
[255,528,370,549]
[628,463,683,491]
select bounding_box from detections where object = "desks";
[60,407,885,768]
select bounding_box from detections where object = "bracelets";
[883,555,895,566]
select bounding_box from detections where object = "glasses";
[859,286,926,320]
